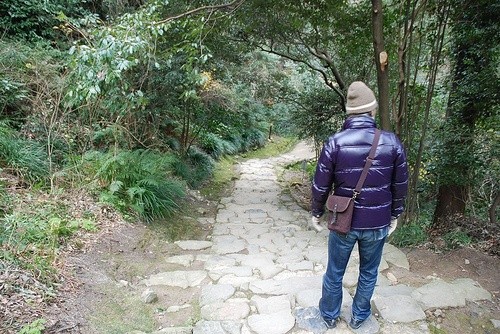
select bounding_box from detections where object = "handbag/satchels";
[324,194,357,235]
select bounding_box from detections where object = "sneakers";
[350,311,371,331]
[318,298,336,328]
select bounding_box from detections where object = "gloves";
[386,217,399,237]
[312,216,323,232]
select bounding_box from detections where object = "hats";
[346,80,378,114]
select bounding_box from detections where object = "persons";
[309,80,409,330]
